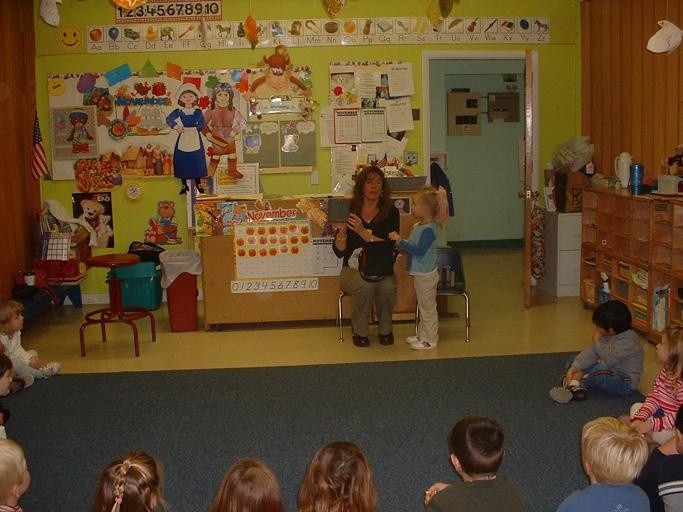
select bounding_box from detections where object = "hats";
[174,83,202,99]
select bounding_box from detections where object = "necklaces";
[363,216,373,224]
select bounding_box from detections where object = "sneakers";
[549,385,588,403]
[9,361,59,393]
[406,335,438,349]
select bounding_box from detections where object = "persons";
[166,82,213,194]
[0,300,60,512]
[296,441,379,512]
[202,83,246,179]
[423,414,528,512]
[87,450,166,512]
[549,300,683,512]
[207,456,286,512]
[332,166,401,347]
[388,185,450,349]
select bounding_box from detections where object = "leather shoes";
[379,332,394,345]
[196,183,205,193]
[351,325,369,347]
[180,185,189,194]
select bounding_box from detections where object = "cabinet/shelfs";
[543,212,582,298]
[581,185,683,344]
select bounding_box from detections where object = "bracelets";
[336,234,348,242]
[369,235,376,242]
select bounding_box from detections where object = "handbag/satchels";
[362,241,394,276]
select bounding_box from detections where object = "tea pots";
[612,150,633,189]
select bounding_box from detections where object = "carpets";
[1,352,663,512]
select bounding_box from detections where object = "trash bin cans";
[159,249,200,332]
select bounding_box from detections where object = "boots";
[228,157,243,179]
[203,160,219,178]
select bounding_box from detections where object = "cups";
[606,176,614,186]
[14,269,51,290]
[632,185,642,197]
[629,163,643,187]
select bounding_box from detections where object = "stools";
[79,254,156,356]
[335,289,376,342]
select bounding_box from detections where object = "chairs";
[415,251,470,342]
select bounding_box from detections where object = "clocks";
[125,183,143,200]
